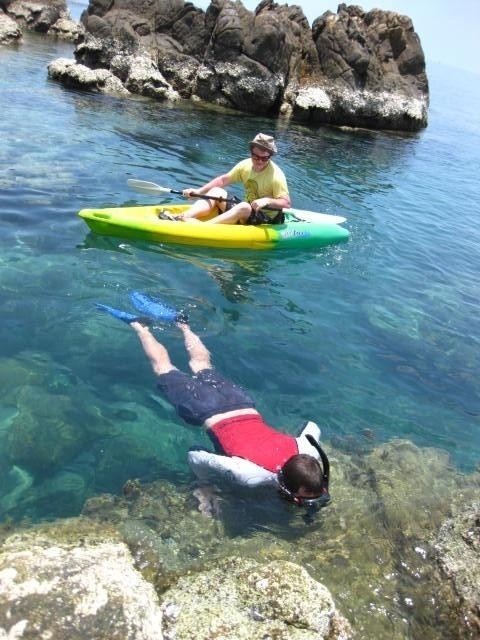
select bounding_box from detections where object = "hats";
[248,133,278,153]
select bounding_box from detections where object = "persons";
[157,131,292,228]
[96,291,330,511]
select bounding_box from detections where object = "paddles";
[127,179,347,224]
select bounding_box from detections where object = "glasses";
[250,149,271,161]
[279,468,330,510]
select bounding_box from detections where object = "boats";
[78,202,353,255]
